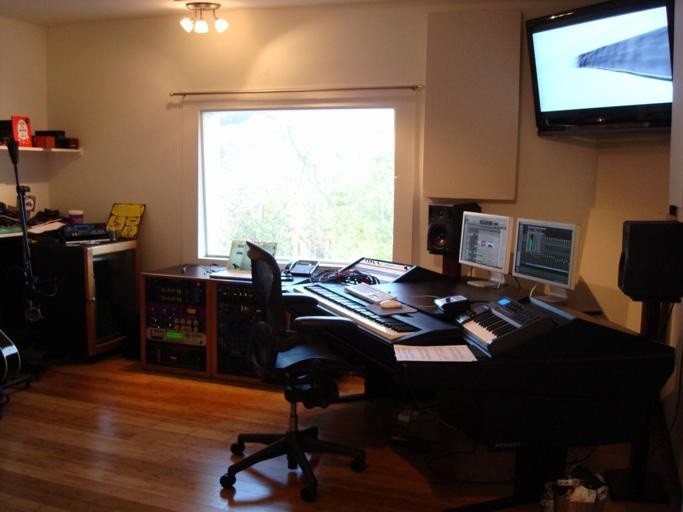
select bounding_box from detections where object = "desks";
[284,277,676,512]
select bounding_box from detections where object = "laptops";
[209,240,277,280]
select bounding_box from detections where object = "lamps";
[179,2,228,34]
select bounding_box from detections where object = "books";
[59,222,112,245]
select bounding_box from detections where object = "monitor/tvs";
[524,0,674,136]
[458,211,513,288]
[511,218,581,304]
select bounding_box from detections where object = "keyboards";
[345,281,397,305]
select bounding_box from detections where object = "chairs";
[221,242,366,501]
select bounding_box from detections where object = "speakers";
[616,220,683,303]
[427,201,482,257]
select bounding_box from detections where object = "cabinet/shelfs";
[0,230,62,346]
[38,240,141,362]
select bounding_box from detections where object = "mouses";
[380,300,402,309]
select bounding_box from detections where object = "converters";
[669,205,677,216]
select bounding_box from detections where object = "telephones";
[284,259,321,277]
